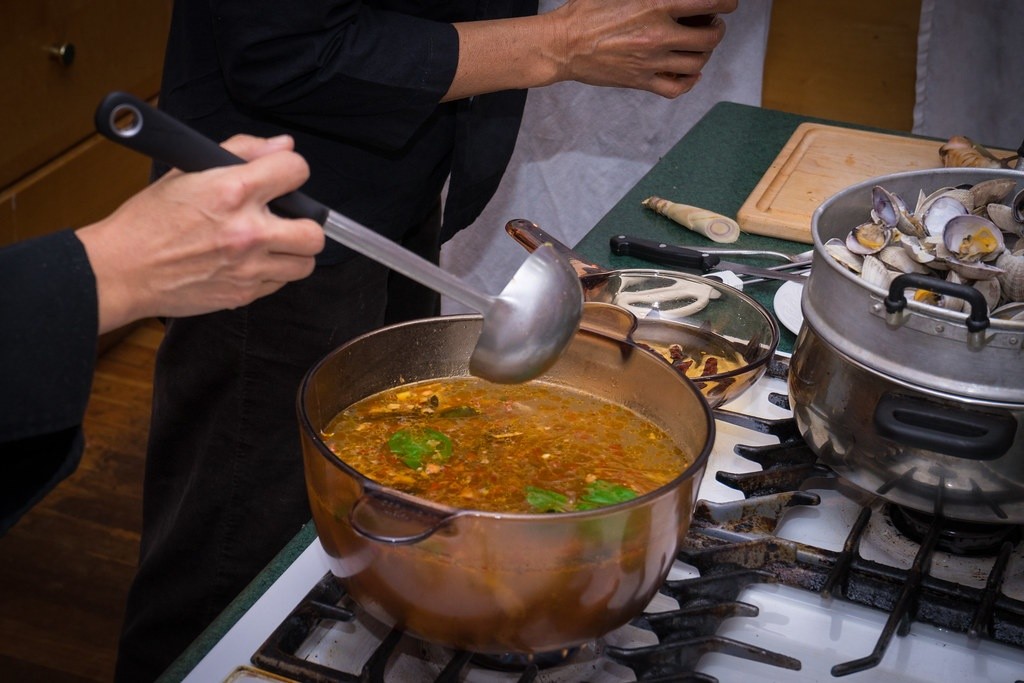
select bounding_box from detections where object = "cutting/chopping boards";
[735,121,1018,245]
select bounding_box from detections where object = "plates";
[773,271,808,335]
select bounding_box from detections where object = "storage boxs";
[296,302,717,655]
[788,169,1023,533]
[505,218,781,412]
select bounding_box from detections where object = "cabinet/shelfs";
[1,1,177,354]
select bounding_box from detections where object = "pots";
[293,299,716,652]
[789,140,1024,527]
[506,212,781,409]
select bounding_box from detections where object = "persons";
[112,1,740,681]
[0,133,325,541]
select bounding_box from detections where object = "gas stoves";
[249,341,1023,682]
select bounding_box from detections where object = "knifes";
[611,233,811,285]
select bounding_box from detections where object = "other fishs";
[937,135,1020,170]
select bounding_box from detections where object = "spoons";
[680,244,815,265]
